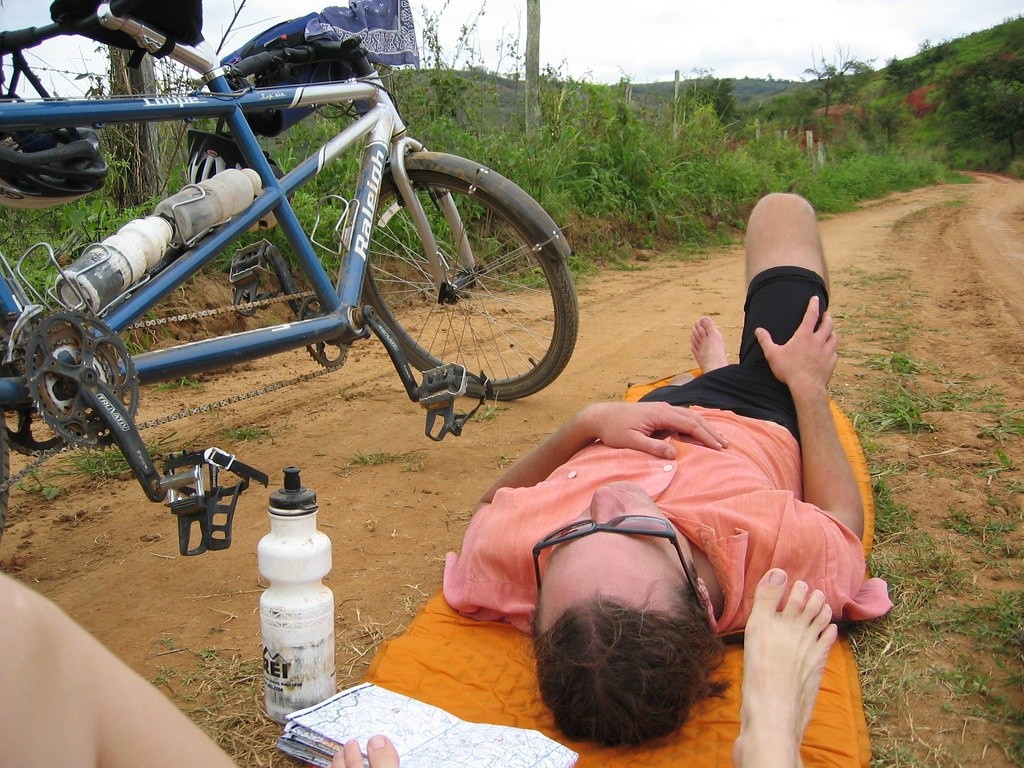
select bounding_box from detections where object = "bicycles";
[0,0,578,560]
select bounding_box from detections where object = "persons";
[442,193,893,746]
[0,568,839,768]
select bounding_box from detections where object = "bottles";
[155,168,262,244]
[257,465,337,724]
[55,215,177,317]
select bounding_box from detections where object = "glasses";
[532,515,701,611]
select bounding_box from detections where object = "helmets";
[0,126,109,209]
[187,129,292,231]
